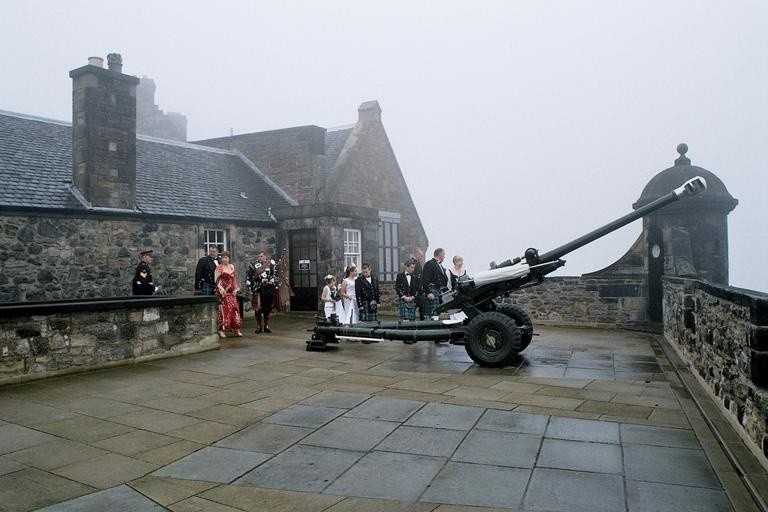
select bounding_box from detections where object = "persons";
[132,250,159,295]
[421,248,448,346]
[413,248,426,320]
[193,244,222,295]
[214,250,244,337]
[245,251,280,333]
[446,254,470,325]
[395,257,419,344]
[339,262,358,342]
[355,263,379,343]
[320,274,335,321]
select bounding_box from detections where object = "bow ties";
[406,273,412,276]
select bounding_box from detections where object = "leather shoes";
[264,326,272,333]
[255,328,262,333]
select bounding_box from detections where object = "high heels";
[234,329,243,337]
[218,330,227,338]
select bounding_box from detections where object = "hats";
[140,250,155,258]
